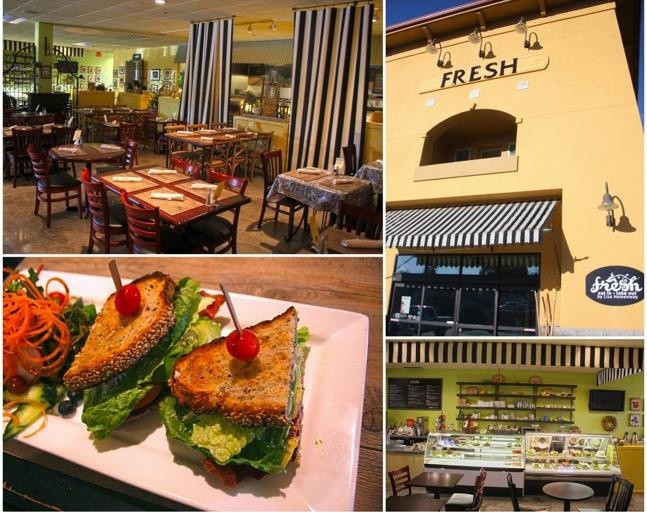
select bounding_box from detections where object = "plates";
[529,375,542,384]
[492,374,505,383]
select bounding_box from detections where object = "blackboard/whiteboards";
[388,377,442,409]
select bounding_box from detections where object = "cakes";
[528,448,609,470]
[569,437,601,447]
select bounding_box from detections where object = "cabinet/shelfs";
[455,382,578,433]
[385,432,644,497]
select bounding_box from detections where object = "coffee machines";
[416,416,429,436]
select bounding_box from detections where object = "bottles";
[332,163,339,177]
[207,187,214,207]
[73,135,83,149]
[387,422,397,435]
[623,432,639,446]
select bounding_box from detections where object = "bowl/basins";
[462,427,477,434]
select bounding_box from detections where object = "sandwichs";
[159,283,310,487]
[66,260,225,441]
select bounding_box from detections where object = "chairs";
[386,465,634,512]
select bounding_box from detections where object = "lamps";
[598,182,618,228]
[426,16,531,68]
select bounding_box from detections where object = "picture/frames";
[112,66,126,89]
[80,66,102,83]
[150,69,171,94]
[630,398,641,427]
